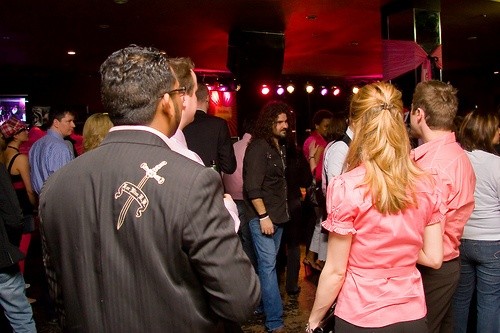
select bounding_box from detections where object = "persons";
[301,109,359,284]
[242,101,296,333]
[445,109,499,333]
[223,121,261,277]
[404,81,477,332]
[82,112,117,158]
[1,163,40,333]
[29,102,80,324]
[163,56,242,234]
[305,81,446,333]
[1,109,93,160]
[2,115,39,305]
[182,82,237,193]
[40,44,261,333]
[275,142,315,296]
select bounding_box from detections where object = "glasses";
[158,87,187,99]
[14,126,29,135]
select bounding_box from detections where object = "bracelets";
[259,212,269,219]
[306,321,325,333]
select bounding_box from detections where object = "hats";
[0,114,27,140]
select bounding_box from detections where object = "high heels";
[303,257,322,278]
